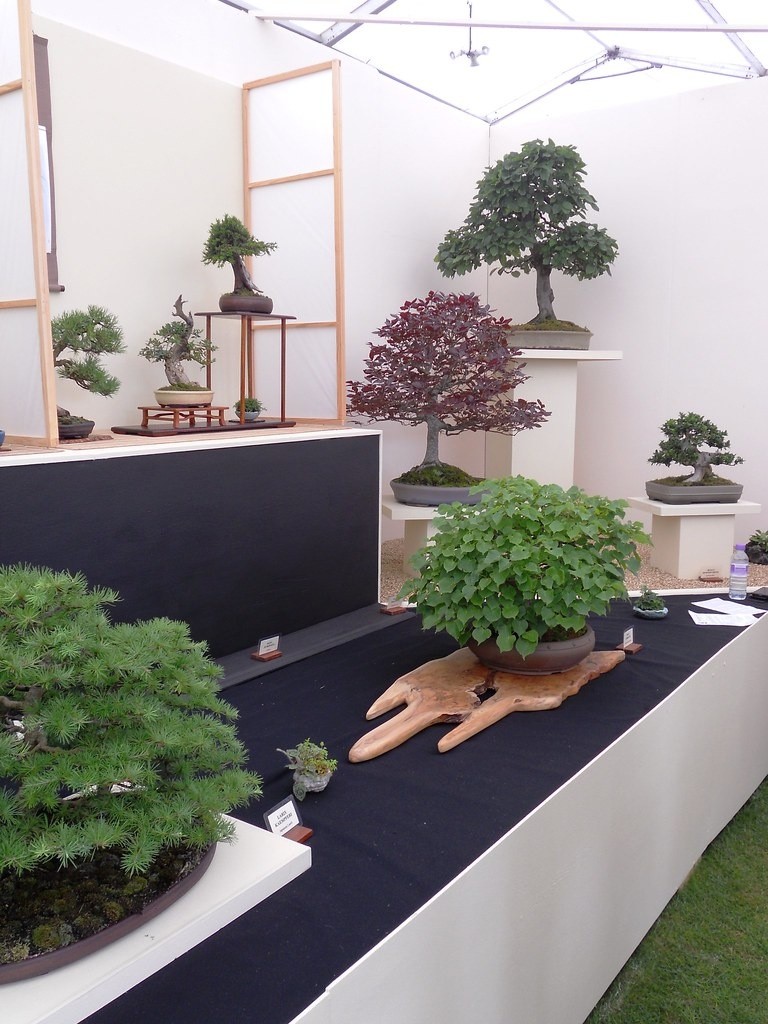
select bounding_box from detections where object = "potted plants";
[345,292,553,504]
[201,212,279,314]
[645,411,746,506]
[233,398,268,421]
[139,294,220,407]
[430,135,619,348]
[634,584,669,620]
[273,735,338,801]
[50,303,129,439]
[0,560,266,987]
[389,474,656,678]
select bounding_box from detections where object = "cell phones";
[749,587,768,600]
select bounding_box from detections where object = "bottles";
[728,544,749,600]
